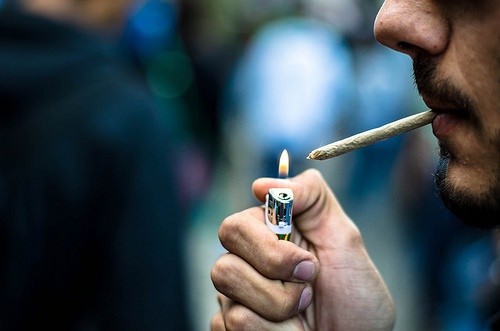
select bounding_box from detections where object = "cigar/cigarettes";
[309,109,437,160]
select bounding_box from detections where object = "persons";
[1,0,193,330]
[210,0,500,330]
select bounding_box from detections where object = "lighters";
[263,188,293,243]
